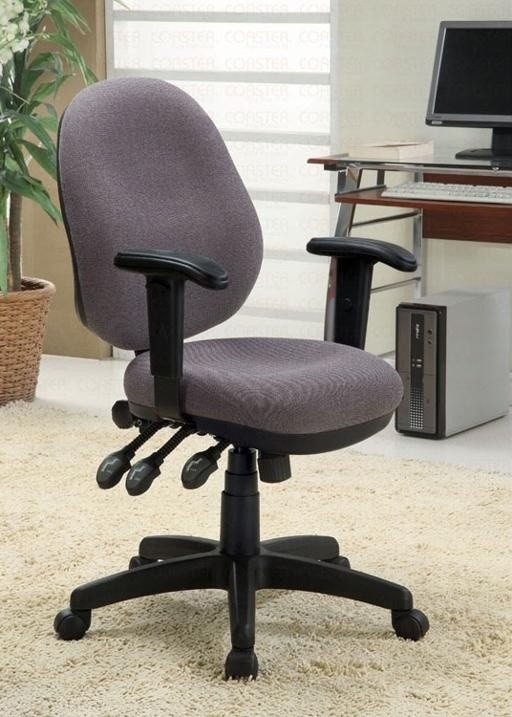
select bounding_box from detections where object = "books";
[349,142,433,160]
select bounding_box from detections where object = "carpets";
[1,399,512,716]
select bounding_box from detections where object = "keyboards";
[380,182,512,205]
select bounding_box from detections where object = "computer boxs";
[396,289,509,440]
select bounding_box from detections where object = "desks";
[307,139,512,345]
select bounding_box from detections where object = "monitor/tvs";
[425,21,512,160]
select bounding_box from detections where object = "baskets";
[0,273,57,409]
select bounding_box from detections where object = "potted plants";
[0,0,99,410]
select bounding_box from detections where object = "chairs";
[53,77,429,680]
